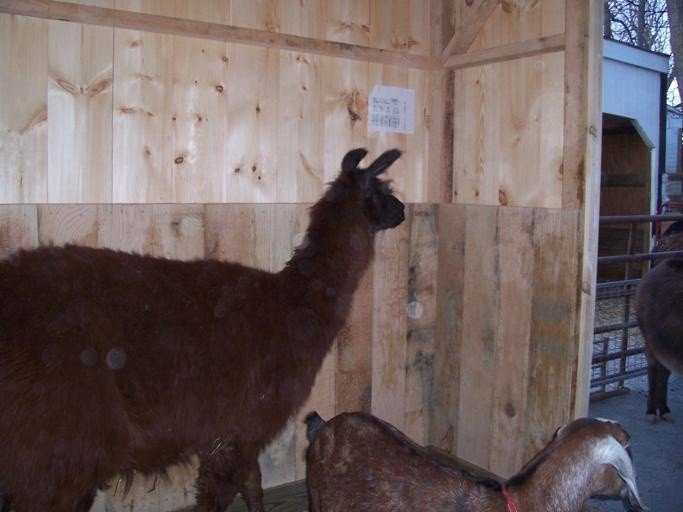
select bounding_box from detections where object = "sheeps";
[305,411,645,512]
[636,257,683,422]
